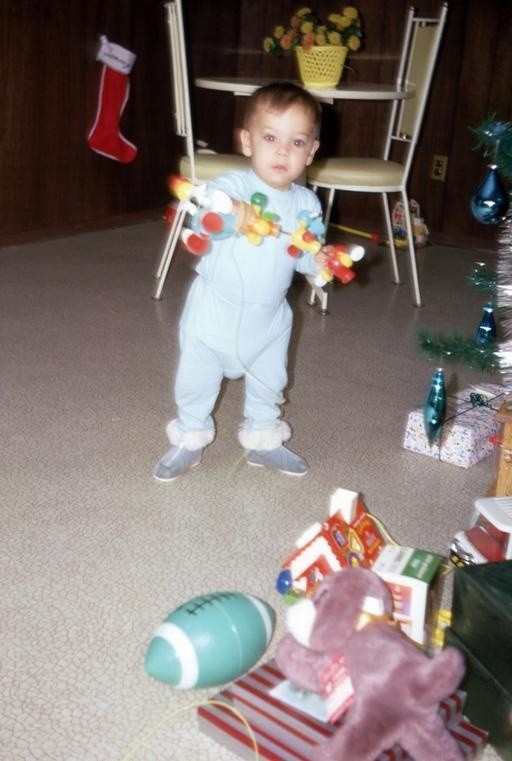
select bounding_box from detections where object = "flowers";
[261,6,366,58]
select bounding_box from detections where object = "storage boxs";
[364,541,447,662]
[446,558,510,685]
[401,382,510,469]
[441,626,512,761]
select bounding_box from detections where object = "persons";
[148,82,335,478]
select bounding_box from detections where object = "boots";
[238,420,311,474]
[152,420,215,483]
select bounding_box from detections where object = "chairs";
[153,0,334,315]
[302,0,451,311]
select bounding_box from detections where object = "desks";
[192,71,416,103]
[489,389,512,499]
